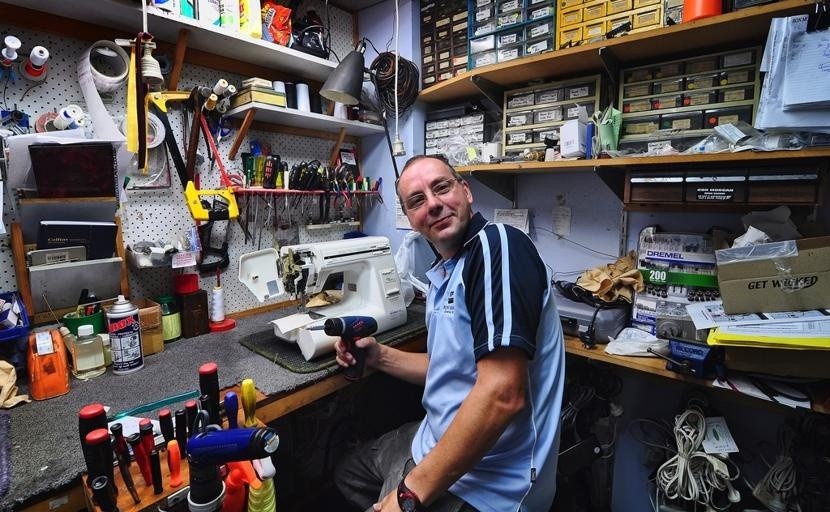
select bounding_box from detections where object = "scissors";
[193,411,223,433]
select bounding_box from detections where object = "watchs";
[397,474,426,512]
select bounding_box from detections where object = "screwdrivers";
[241,140,321,251]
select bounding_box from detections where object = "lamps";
[324,37,402,182]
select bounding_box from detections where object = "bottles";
[286,83,297,109]
[105,291,143,378]
[681,1,724,24]
[296,84,311,112]
[273,81,287,108]
[154,294,183,343]
[60,326,111,380]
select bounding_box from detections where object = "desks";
[4,297,829,512]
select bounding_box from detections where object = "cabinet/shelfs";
[2,0,385,138]
[621,165,824,215]
[421,0,828,174]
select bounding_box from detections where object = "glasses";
[401,177,460,212]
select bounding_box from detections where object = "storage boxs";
[712,228,830,313]
[553,288,629,346]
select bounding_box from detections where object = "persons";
[333,156,567,511]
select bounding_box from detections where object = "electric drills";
[306,316,377,382]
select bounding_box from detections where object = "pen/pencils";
[75,304,102,318]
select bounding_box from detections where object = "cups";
[309,95,322,114]
[63,309,105,336]
